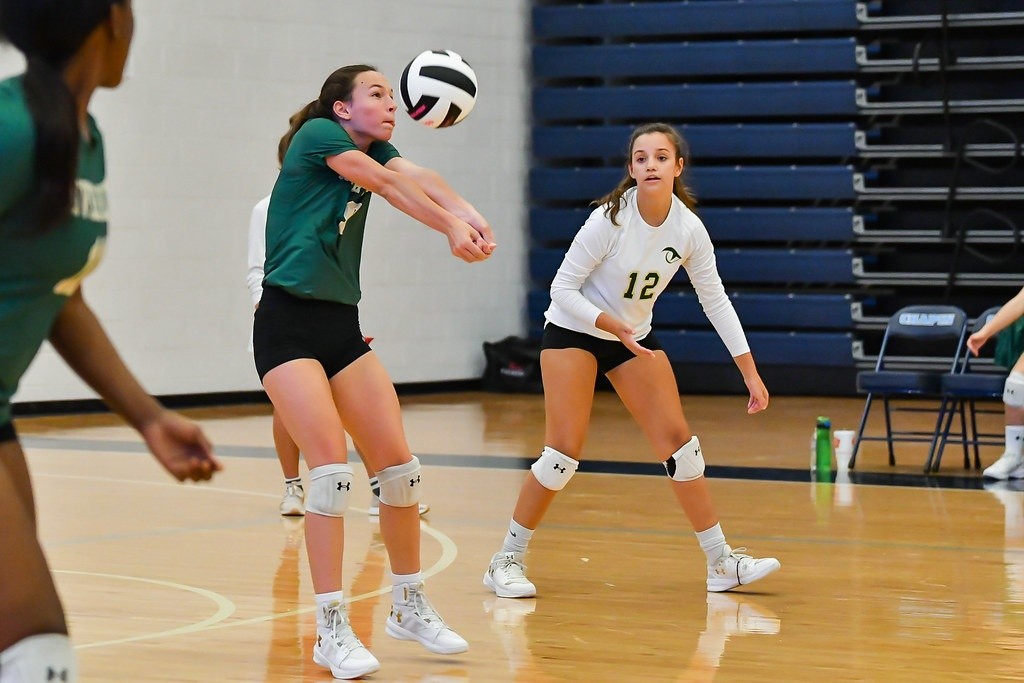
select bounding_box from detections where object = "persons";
[254,65,494,679]
[0,0,222,683]
[483,123,780,598]
[967,286,1024,479]
[485,592,781,683]
[247,132,307,515]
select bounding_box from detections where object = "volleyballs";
[398,48,478,129]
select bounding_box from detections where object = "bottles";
[810,428,817,470]
[816,417,831,469]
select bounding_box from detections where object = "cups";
[833,430,856,468]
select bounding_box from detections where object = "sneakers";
[281,481,306,515]
[482,597,536,622]
[384,579,469,654]
[706,593,781,636]
[368,492,430,515]
[313,599,382,680]
[706,546,781,593]
[983,451,1024,480]
[483,551,538,598]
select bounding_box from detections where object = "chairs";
[848,305,970,472]
[932,307,1005,472]
